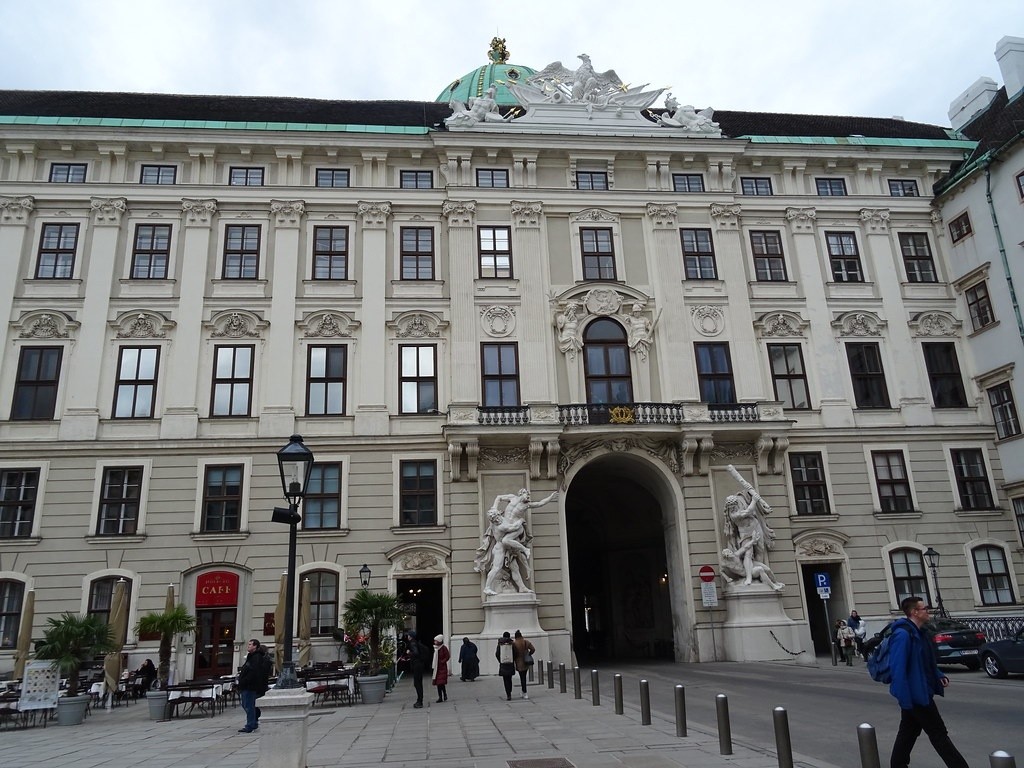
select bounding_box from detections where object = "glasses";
[915,608,928,613]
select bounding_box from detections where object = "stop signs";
[698,565,715,582]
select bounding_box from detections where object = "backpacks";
[411,640,429,660]
[868,626,913,684]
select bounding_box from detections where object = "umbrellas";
[274,572,287,677]
[103,577,129,713]
[298,577,311,669]
[11,587,36,680]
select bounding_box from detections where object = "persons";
[404,631,423,708]
[848,609,861,643]
[723,495,768,586]
[584,77,598,92]
[495,632,519,700]
[514,629,535,699]
[571,81,584,102]
[483,488,559,596]
[447,87,499,122]
[238,639,270,733]
[890,597,969,768]
[718,531,785,590]
[432,634,450,703]
[854,620,866,659]
[477,508,532,581]
[837,620,855,666]
[132,659,156,699]
[832,620,846,662]
[458,637,479,682]
[664,96,680,109]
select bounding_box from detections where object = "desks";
[164,669,355,722]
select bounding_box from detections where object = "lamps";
[358,564,372,591]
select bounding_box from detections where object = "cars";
[980,626,1024,680]
[862,617,986,673]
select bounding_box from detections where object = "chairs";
[0,671,149,731]
[180,662,358,719]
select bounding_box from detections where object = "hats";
[434,634,443,643]
[515,630,522,639]
[406,631,416,637]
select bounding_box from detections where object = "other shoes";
[519,692,528,699]
[238,724,259,733]
[460,677,466,682]
[839,659,846,662]
[436,699,443,703]
[414,702,423,708]
[444,695,447,700]
[849,663,852,666]
[471,679,475,682]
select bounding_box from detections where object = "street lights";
[273,433,315,688]
[923,545,945,619]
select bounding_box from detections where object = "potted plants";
[341,585,408,705]
[33,609,122,728]
[130,583,201,720]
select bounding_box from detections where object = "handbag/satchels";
[523,640,534,665]
[844,639,852,647]
